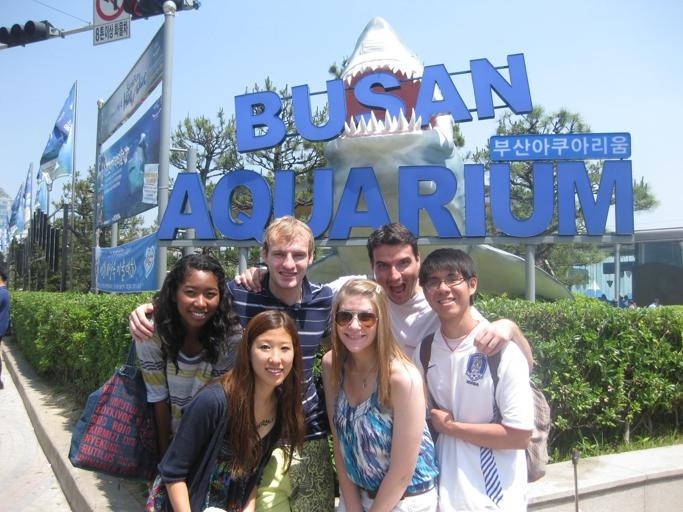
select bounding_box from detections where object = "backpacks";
[419,332,552,483]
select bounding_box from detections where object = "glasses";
[334,311,380,328]
[422,277,470,292]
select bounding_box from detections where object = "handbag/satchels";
[69,338,159,484]
[5,321,14,337]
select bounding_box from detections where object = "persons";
[128,214,336,512]
[145,310,306,512]
[135,254,245,460]
[408,246,533,512]
[233,221,535,440]
[321,277,440,512]
[0,273,10,389]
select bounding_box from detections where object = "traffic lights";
[0,21,47,48]
[122,0,184,22]
